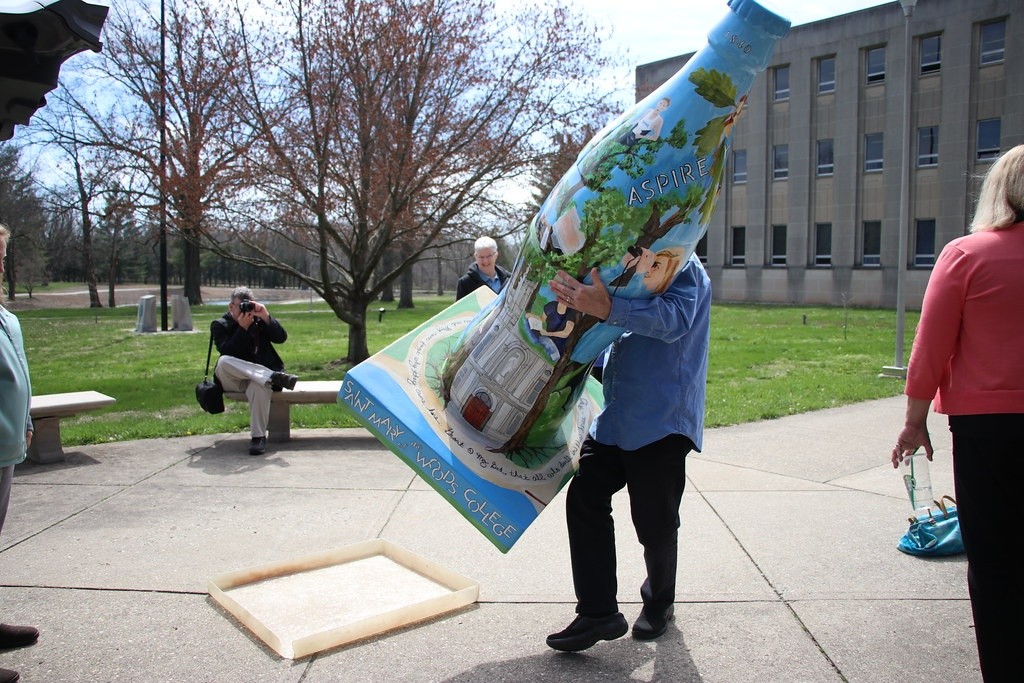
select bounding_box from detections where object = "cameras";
[239,299,255,315]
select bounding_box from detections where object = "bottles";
[442,0,791,451]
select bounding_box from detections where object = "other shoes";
[0,623,40,654]
[249,436,266,455]
[0,668,19,683]
[273,372,298,391]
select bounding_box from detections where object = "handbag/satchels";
[897,495,966,556]
[195,380,225,414]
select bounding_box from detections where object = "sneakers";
[631,604,675,640]
[546,612,628,651]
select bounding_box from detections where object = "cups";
[896,453,934,510]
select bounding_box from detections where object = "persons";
[892,144,1024,683]
[456,237,512,302]
[0,226,40,683]
[546,251,713,652]
[211,287,298,454]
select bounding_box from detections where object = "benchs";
[28,390,117,465]
[224,380,343,443]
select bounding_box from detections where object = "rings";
[567,297,572,303]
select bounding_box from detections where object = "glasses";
[475,251,497,259]
